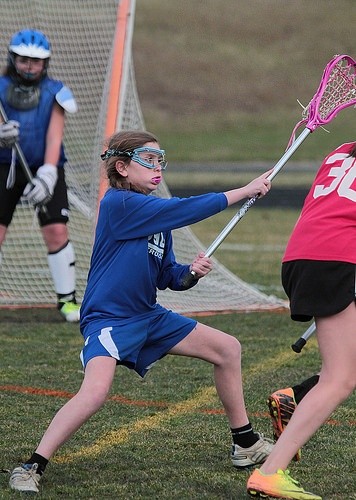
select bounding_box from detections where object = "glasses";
[106,147,168,170]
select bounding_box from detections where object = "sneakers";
[9,467,44,492]
[245,467,322,500]
[56,297,82,322]
[267,388,302,462]
[230,432,277,467]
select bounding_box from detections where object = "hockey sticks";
[179,53,356,289]
[292,322,316,353]
[0,99,48,214]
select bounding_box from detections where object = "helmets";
[8,29,51,58]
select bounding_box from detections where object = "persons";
[0,28,83,323]
[9,131,275,492]
[245,144,356,500]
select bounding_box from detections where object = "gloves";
[0,120,21,149]
[22,164,59,207]
